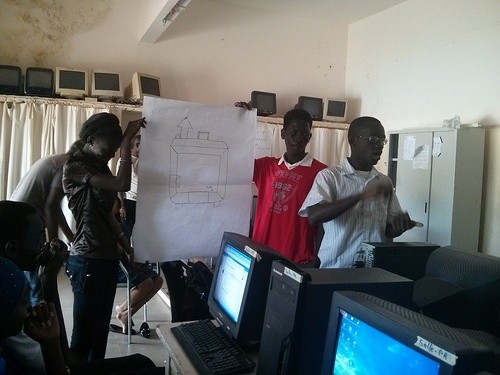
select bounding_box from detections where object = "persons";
[8,139,68,243]
[115,135,142,245]
[298,116,424,268]
[1,200,160,375]
[234,101,328,267]
[111,198,164,335]
[61,114,147,364]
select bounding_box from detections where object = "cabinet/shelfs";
[385,126,487,254]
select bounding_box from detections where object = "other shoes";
[109,324,136,335]
[140,322,150,338]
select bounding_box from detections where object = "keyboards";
[171,319,256,375]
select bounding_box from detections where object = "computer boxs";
[256,242,442,375]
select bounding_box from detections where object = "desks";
[153,317,258,375]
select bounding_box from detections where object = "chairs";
[116,247,148,344]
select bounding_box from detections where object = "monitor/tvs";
[207,232,301,353]
[323,97,348,123]
[90,68,124,102]
[124,72,163,104]
[294,96,323,121]
[0,65,22,96]
[24,67,54,98]
[55,67,88,99]
[249,91,276,117]
[321,246,500,375]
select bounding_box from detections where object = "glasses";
[353,136,388,145]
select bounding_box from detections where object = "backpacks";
[179,260,214,304]
